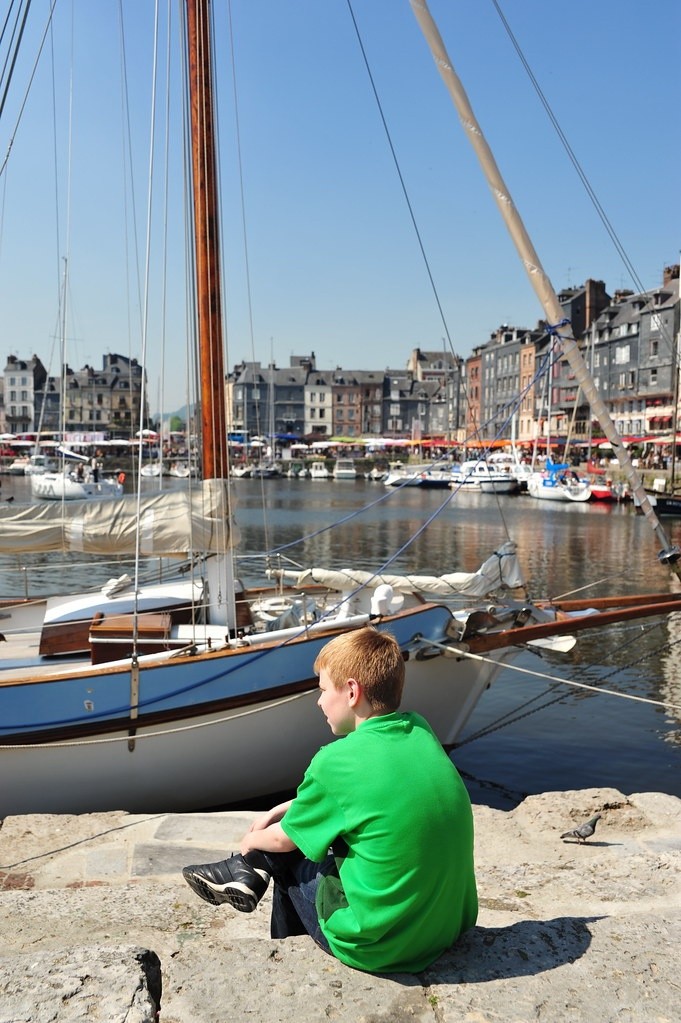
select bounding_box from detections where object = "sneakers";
[182,852,272,914]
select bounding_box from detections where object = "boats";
[0,1,681,816]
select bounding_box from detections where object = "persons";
[183,625,479,974]
[77,462,84,482]
[627,447,670,470]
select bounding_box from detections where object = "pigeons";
[560,813,606,845]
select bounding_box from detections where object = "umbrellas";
[135,429,157,436]
[229,431,681,449]
